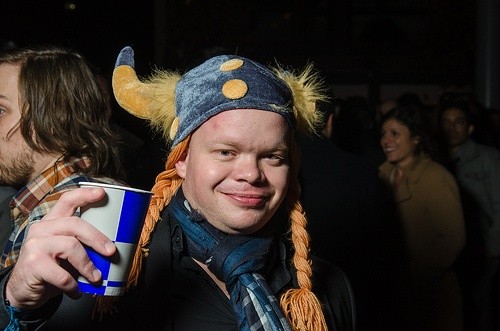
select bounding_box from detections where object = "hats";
[111,45,331,331]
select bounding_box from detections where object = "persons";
[298,85,500,331]
[0,46,355,331]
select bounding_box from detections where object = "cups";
[77,182,155,297]
